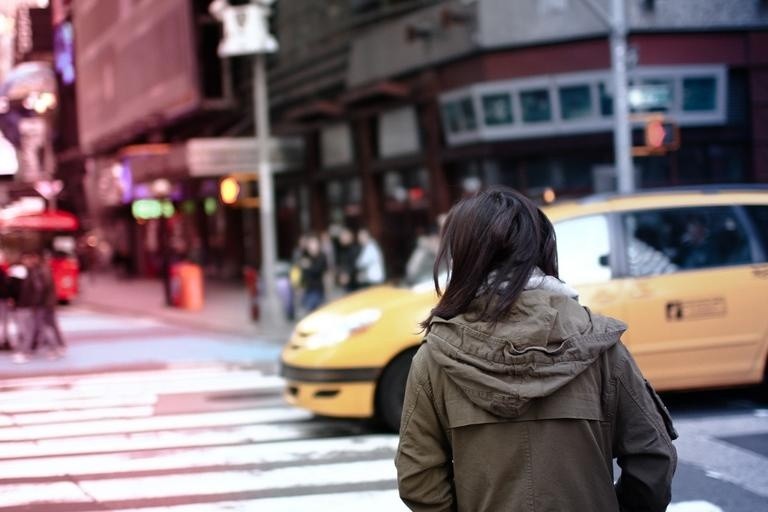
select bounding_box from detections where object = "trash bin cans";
[166,261,204,311]
[47,250,79,305]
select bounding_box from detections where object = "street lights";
[206,0,287,340]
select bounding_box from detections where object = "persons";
[395,185,678,512]
[0,250,67,361]
[293,203,388,315]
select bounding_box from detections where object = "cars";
[279,188,768,433]
